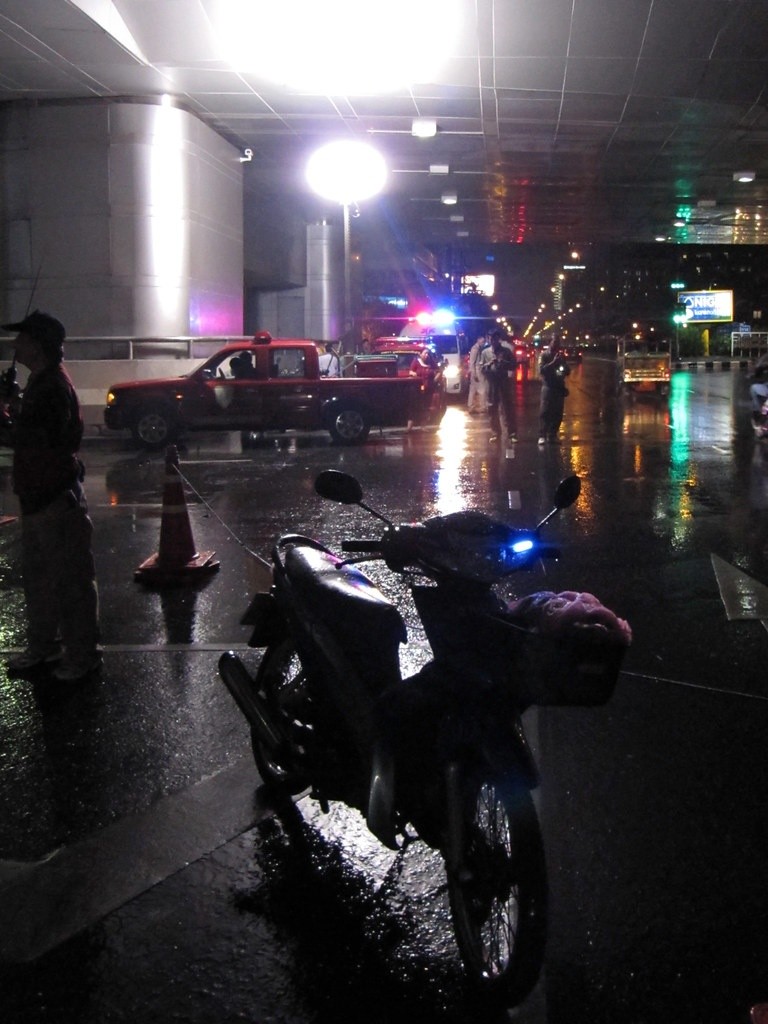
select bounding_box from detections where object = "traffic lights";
[672,303,687,323]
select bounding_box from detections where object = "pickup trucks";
[104,330,433,457]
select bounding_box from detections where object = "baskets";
[476,606,629,706]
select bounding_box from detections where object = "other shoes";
[56,648,104,683]
[510,436,519,443]
[538,435,547,445]
[489,434,499,442]
[549,436,562,444]
[6,642,66,670]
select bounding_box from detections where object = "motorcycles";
[218,469,634,1014]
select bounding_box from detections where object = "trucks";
[617,336,670,399]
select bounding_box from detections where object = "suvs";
[369,336,449,428]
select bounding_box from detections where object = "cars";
[563,346,583,362]
[513,339,530,364]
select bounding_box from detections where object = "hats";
[0,309,66,346]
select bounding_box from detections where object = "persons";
[361,338,373,355]
[318,344,339,377]
[750,383,768,431]
[406,348,442,432]
[0,310,105,671]
[537,332,570,444]
[466,329,529,443]
[229,351,256,379]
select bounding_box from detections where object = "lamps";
[430,164,449,175]
[670,218,685,227]
[655,235,667,241]
[440,195,457,204]
[450,216,464,222]
[412,120,437,138]
[697,200,716,210]
[733,173,755,182]
[456,231,469,237]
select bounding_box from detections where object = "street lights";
[671,282,684,360]
[531,286,608,337]
[492,304,515,337]
[302,137,393,352]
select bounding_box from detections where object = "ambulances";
[398,310,470,394]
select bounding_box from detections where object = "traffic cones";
[134,443,222,580]
